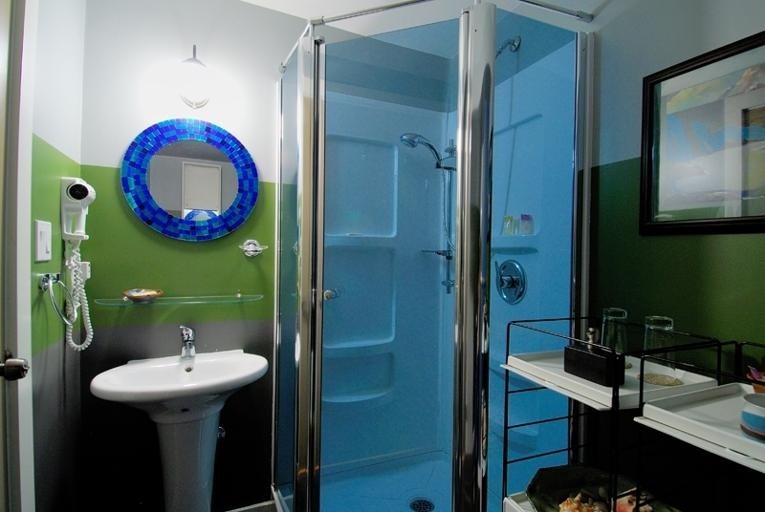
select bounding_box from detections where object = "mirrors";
[119,117,259,243]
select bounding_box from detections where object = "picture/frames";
[638,29,764,234]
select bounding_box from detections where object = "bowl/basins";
[742,394,765,441]
[121,288,164,304]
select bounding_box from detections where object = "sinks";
[90,347,270,411]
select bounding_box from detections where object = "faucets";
[179,325,196,358]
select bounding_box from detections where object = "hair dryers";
[67,180,96,250]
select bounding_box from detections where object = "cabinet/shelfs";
[501,317,725,511]
[634,340,765,512]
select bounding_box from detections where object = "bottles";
[602,306,676,387]
